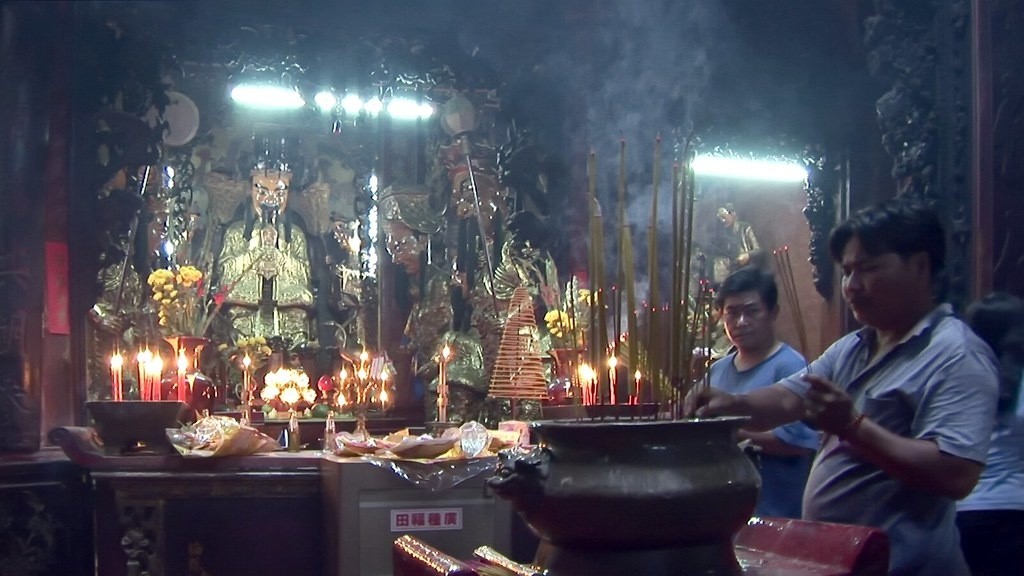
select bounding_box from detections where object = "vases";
[543,347,586,403]
[239,371,253,412]
[163,335,215,418]
[290,409,299,452]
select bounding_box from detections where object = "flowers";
[148,265,228,338]
[262,366,317,406]
[217,338,271,373]
[545,286,597,343]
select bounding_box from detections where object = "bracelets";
[838,413,866,441]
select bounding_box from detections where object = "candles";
[440,361,445,386]
[111,350,186,401]
[243,372,250,390]
[580,355,641,405]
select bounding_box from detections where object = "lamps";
[339,351,388,437]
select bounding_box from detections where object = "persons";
[67,188,142,336]
[217,165,320,347]
[328,212,364,307]
[717,201,760,274]
[386,212,453,398]
[439,141,558,333]
[702,263,820,520]
[690,298,737,377]
[955,292,1024,576]
[684,201,1001,576]
